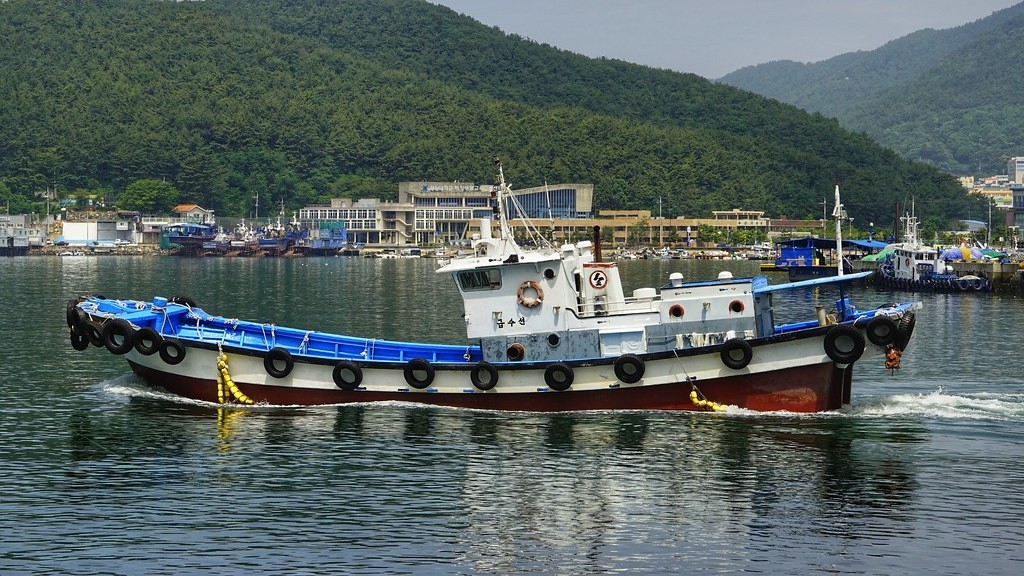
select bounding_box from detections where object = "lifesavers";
[866,315,898,347]
[332,361,364,391]
[470,360,500,391]
[544,362,574,390]
[823,324,866,365]
[263,347,294,379]
[169,294,198,309]
[884,276,992,292]
[403,358,436,390]
[905,258,910,266]
[613,352,647,384]
[894,311,917,352]
[65,293,187,366]
[517,280,545,308]
[720,337,753,370]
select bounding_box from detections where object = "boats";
[231,217,257,250]
[424,250,450,257]
[879,190,993,292]
[788,248,853,282]
[257,216,287,249]
[66,154,925,412]
[203,236,232,252]
